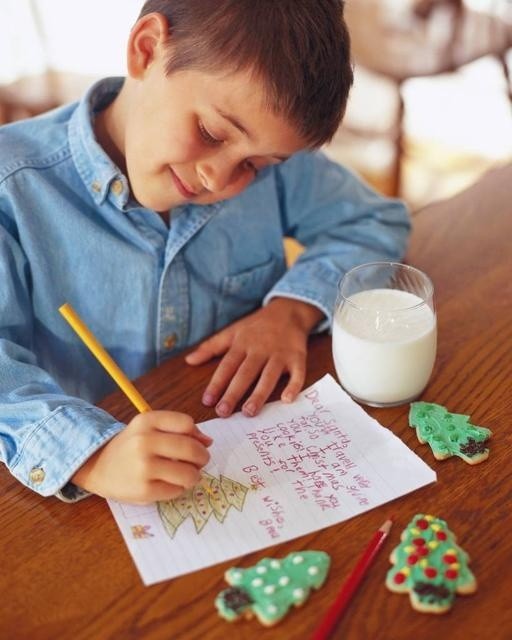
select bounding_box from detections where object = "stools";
[325,0,511,203]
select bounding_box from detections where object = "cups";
[332,261,437,408]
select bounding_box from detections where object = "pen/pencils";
[312,517,395,640]
[60,302,151,415]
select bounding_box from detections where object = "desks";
[0,158,510,638]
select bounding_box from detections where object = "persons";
[0,1,414,502]
